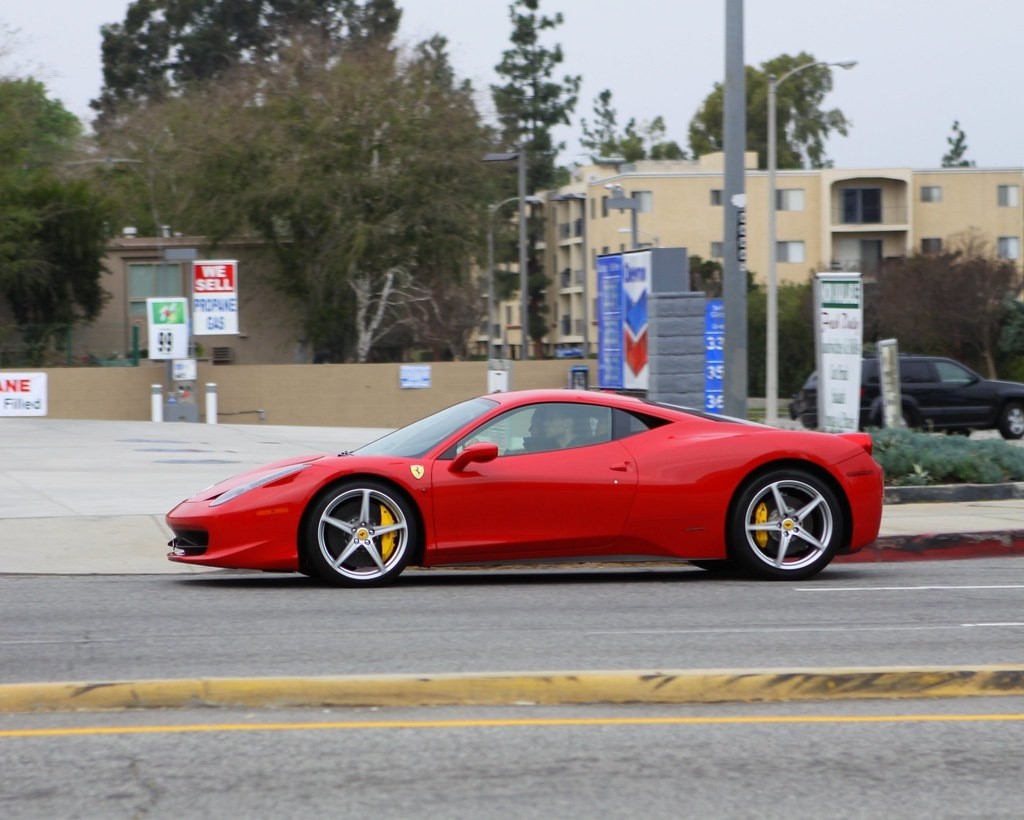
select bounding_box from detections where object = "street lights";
[483,194,547,360]
[763,55,863,426]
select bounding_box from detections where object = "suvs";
[787,354,1024,440]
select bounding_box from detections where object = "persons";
[522,405,600,450]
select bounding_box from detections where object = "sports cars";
[165,385,888,591]
